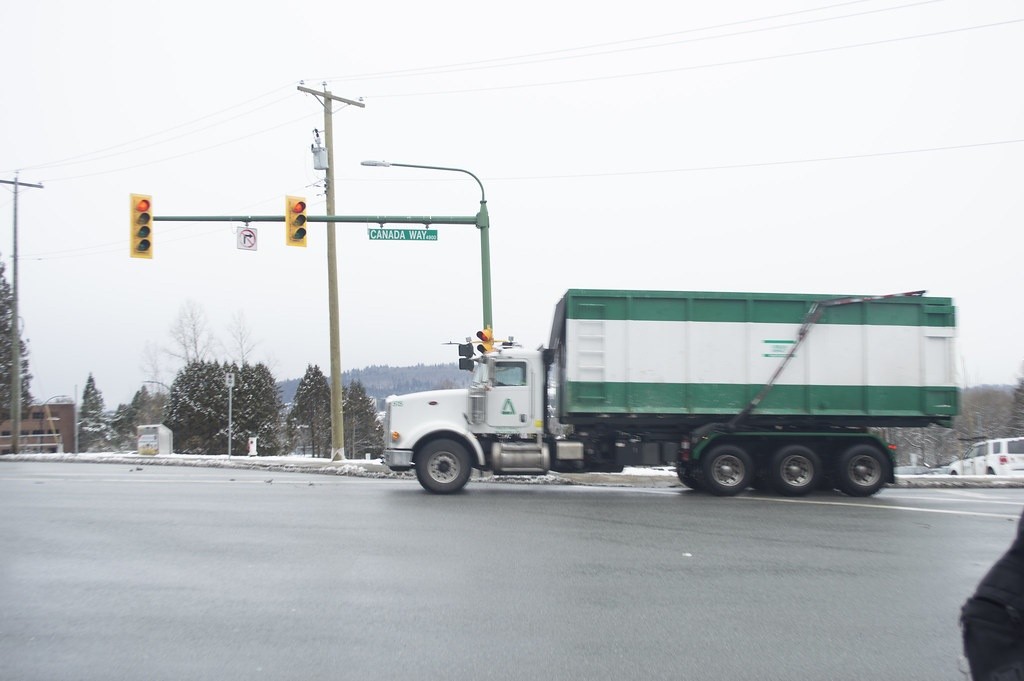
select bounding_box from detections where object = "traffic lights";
[284,195,311,247]
[130,192,154,258]
[473,328,493,357]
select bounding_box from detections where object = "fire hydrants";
[248,431,257,457]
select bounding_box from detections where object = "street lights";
[366,159,494,332]
[38,395,67,453]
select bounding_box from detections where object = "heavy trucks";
[378,288,960,504]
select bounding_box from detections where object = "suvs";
[946,433,1024,479]
